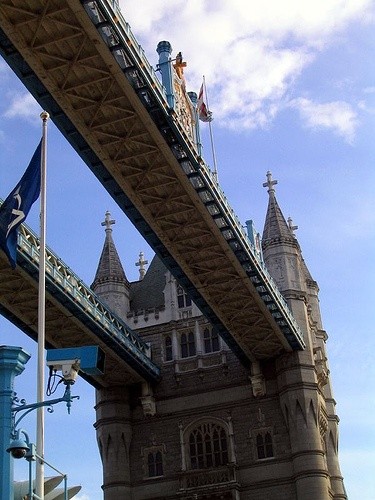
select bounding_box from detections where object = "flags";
[198,86,213,122]
[0,135,42,271]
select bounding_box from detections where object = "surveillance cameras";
[46,345,105,384]
[6,439,30,459]
